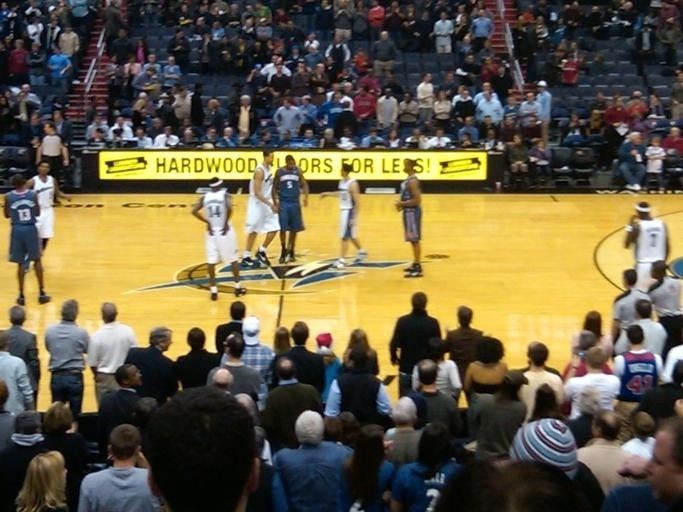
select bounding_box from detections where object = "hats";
[316,333,333,350]
[456,68,468,76]
[241,316,260,345]
[48,5,56,12]
[508,417,579,479]
[537,80,548,86]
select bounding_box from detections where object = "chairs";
[510,0,683,188]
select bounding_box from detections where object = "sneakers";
[38,296,51,304]
[241,257,260,267]
[255,248,271,266]
[16,297,24,305]
[211,292,218,300]
[279,248,288,263]
[404,262,423,278]
[332,257,346,269]
[352,249,367,264]
[235,288,247,297]
[288,248,296,261]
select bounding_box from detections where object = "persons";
[609,268,660,358]
[20,158,73,277]
[316,163,367,270]
[0,290,683,512]
[236,148,280,270]
[0,1,683,205]
[624,202,671,291]
[189,177,247,301]
[2,172,51,305]
[393,159,423,278]
[270,154,310,265]
[645,259,682,364]
